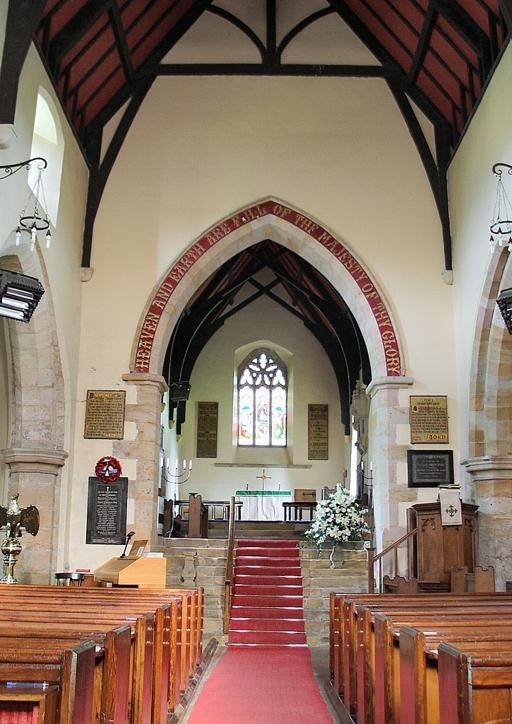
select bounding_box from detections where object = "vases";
[330,545,345,569]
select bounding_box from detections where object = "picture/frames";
[410,395,449,445]
[84,389,126,440]
[407,450,454,488]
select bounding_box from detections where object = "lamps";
[496,286,512,337]
[0,269,44,323]
[489,162,512,253]
[0,157,51,252]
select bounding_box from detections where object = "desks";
[282,502,317,523]
[177,500,243,520]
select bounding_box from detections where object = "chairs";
[55,572,85,587]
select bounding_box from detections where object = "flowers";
[304,482,372,547]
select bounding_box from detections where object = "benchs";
[329,590,512,724]
[1,586,204,723]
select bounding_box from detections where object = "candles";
[189,460,192,468]
[370,462,373,470]
[361,462,364,470]
[183,459,186,468]
[176,459,178,468]
[167,458,169,467]
[161,458,163,467]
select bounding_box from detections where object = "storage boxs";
[294,489,316,502]
